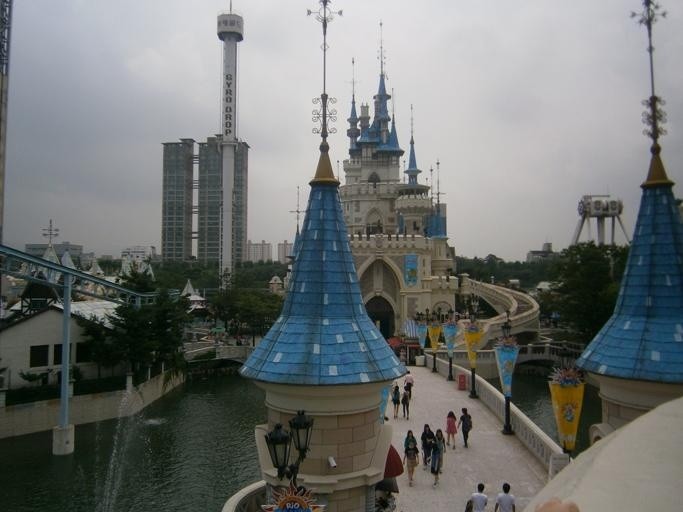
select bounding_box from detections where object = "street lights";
[447,308,455,381]
[432,310,438,373]
[559,344,570,369]
[264,409,314,487]
[469,311,478,398]
[501,321,515,435]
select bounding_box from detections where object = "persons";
[494,482,516,512]
[445,407,473,449]
[404,423,446,487]
[403,370,414,399]
[466,483,488,512]
[393,385,411,420]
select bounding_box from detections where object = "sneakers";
[393,415,409,421]
[446,441,468,450]
[408,462,443,489]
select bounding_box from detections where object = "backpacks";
[402,392,408,404]
